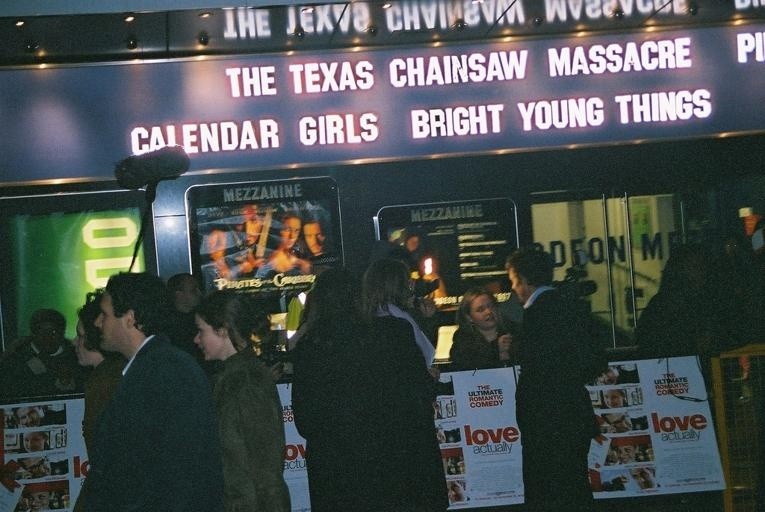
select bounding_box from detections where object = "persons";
[505,244,603,512]
[636,243,741,428]
[289,240,449,512]
[449,286,513,371]
[0,270,292,512]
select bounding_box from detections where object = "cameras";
[415,277,443,299]
